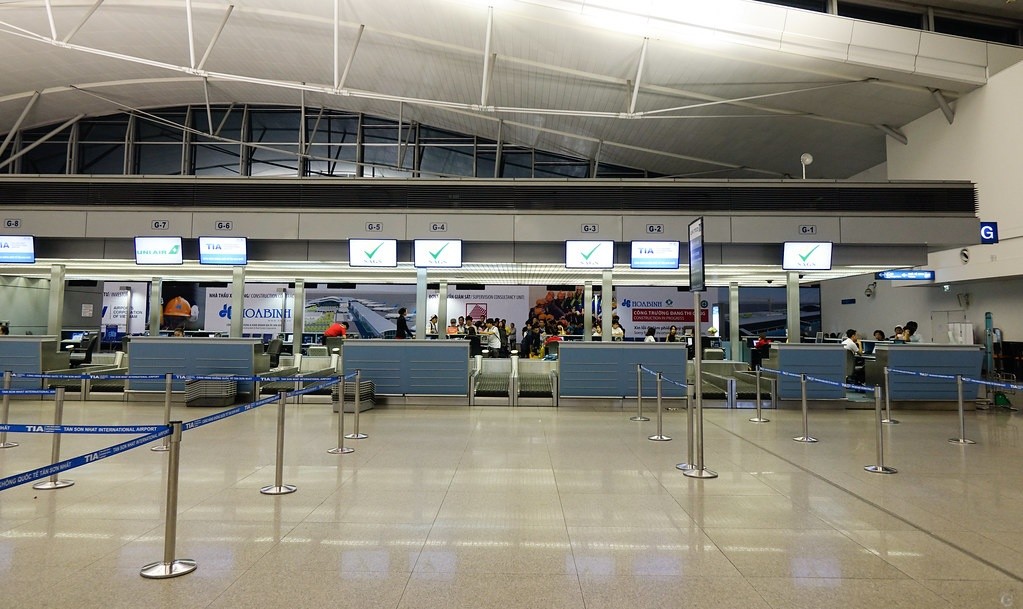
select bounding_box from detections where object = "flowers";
[708,327,718,334]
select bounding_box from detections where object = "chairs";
[69,335,98,367]
[846,349,863,393]
[267,339,284,368]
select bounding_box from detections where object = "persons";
[665,326,682,342]
[425,315,625,359]
[873,321,923,342]
[755,331,772,347]
[322,321,349,346]
[841,329,864,356]
[530,289,617,316]
[643,327,657,343]
[393,307,417,340]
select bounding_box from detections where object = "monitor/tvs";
[198,236,247,265]
[414,239,463,268]
[782,241,833,270]
[348,238,398,267]
[0,234,35,263]
[564,240,614,269]
[134,235,183,265]
[630,240,680,269]
[753,340,759,346]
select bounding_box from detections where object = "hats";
[429,314,436,321]
[894,325,903,330]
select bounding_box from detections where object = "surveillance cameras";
[865,288,872,297]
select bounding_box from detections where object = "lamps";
[801,152,813,179]
